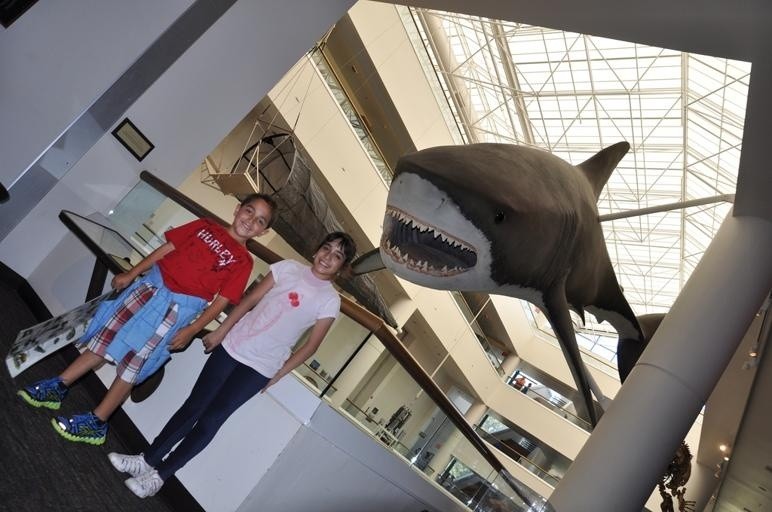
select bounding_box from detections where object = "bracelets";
[219,325,229,335]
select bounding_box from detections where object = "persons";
[23,192,276,445]
[110,231,356,498]
[514,375,525,389]
[521,383,532,393]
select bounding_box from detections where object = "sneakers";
[108,450,155,478]
[124,470,165,498]
[51,409,109,447]
[19,376,68,410]
